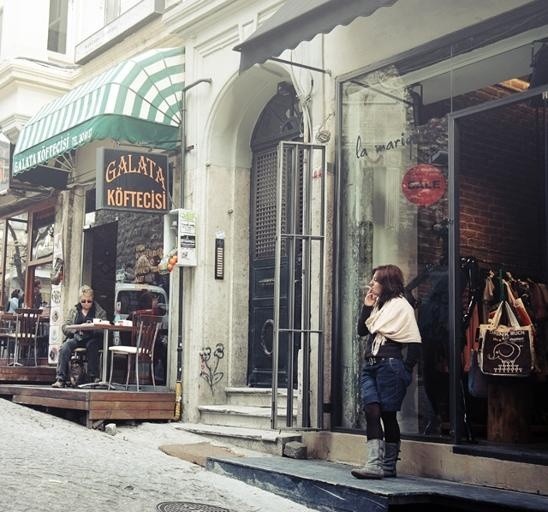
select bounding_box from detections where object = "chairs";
[107,308,167,391]
[0,308,50,367]
[70,346,104,389]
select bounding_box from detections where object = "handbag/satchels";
[5,300,10,312]
[464,296,536,396]
[70,352,89,387]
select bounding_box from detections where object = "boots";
[351,440,384,479]
[382,440,400,477]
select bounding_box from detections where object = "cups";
[92,318,110,324]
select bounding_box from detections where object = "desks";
[64,322,165,393]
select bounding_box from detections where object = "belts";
[367,357,395,365]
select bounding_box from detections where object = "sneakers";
[94,377,102,382]
[52,378,65,388]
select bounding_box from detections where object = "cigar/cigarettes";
[367,286,371,290]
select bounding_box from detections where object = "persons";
[403,226,484,439]
[115,232,164,347]
[51,285,108,389]
[351,263,424,478]
[32,279,42,310]
[7,289,21,315]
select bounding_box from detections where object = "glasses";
[81,299,93,303]
